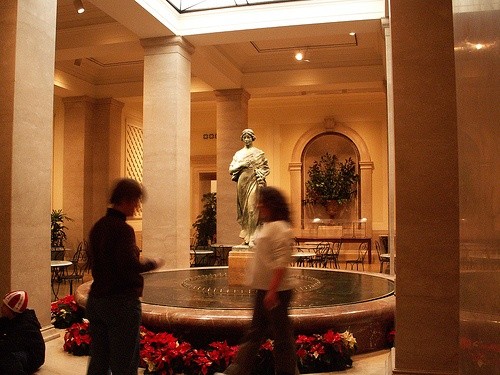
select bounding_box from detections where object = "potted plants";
[51,209,74,261]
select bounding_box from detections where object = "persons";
[215,187,300,375]
[229,129,270,245]
[85,178,164,375]
[0,290,45,375]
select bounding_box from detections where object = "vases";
[73,345,89,356]
[55,320,71,329]
[154,362,176,375]
[184,365,215,375]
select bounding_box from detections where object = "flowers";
[304,152,361,210]
[137,326,357,375]
[63,318,92,351]
[50,294,78,320]
[386,328,395,344]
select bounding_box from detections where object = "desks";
[189,250,214,268]
[211,244,234,267]
[50,260,73,301]
[379,235,396,262]
[290,238,372,267]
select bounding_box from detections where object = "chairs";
[291,238,396,274]
[59,238,89,295]
